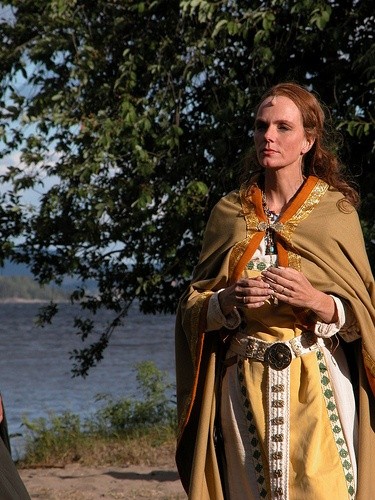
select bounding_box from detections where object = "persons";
[175,83,374,500]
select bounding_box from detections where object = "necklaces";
[262,193,279,305]
